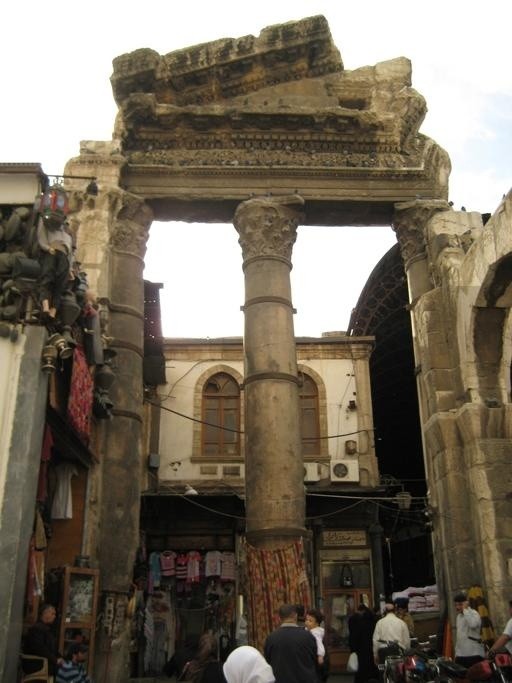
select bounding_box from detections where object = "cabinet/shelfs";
[57,566,100,683]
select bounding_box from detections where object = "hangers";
[149,546,234,555]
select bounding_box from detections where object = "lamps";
[183,484,197,496]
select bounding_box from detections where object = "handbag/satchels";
[346,652,359,673]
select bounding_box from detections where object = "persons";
[294,604,306,628]
[486,598,511,660]
[300,608,327,666]
[21,603,65,675]
[178,633,220,682]
[56,643,91,683]
[36,207,75,325]
[347,597,417,681]
[162,631,199,678]
[447,592,486,668]
[221,645,277,682]
[263,603,330,682]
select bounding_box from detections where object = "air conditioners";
[302,463,320,482]
[331,460,359,482]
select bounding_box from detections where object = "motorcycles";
[378,635,468,683]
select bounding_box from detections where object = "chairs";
[18,653,54,682]
